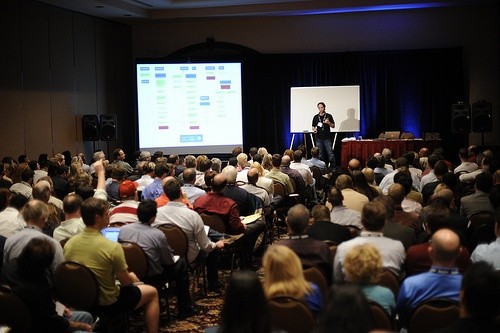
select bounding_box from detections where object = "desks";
[341,139,441,167]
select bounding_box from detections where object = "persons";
[312,102,337,169]
[0,144,500,333]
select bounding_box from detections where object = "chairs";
[0,182,495,333]
[379,131,414,139]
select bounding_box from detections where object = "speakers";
[82,115,99,141]
[451,103,471,135]
[100,113,118,141]
[471,101,494,132]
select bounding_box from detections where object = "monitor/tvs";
[101,227,119,242]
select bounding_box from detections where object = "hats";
[120,180,138,197]
